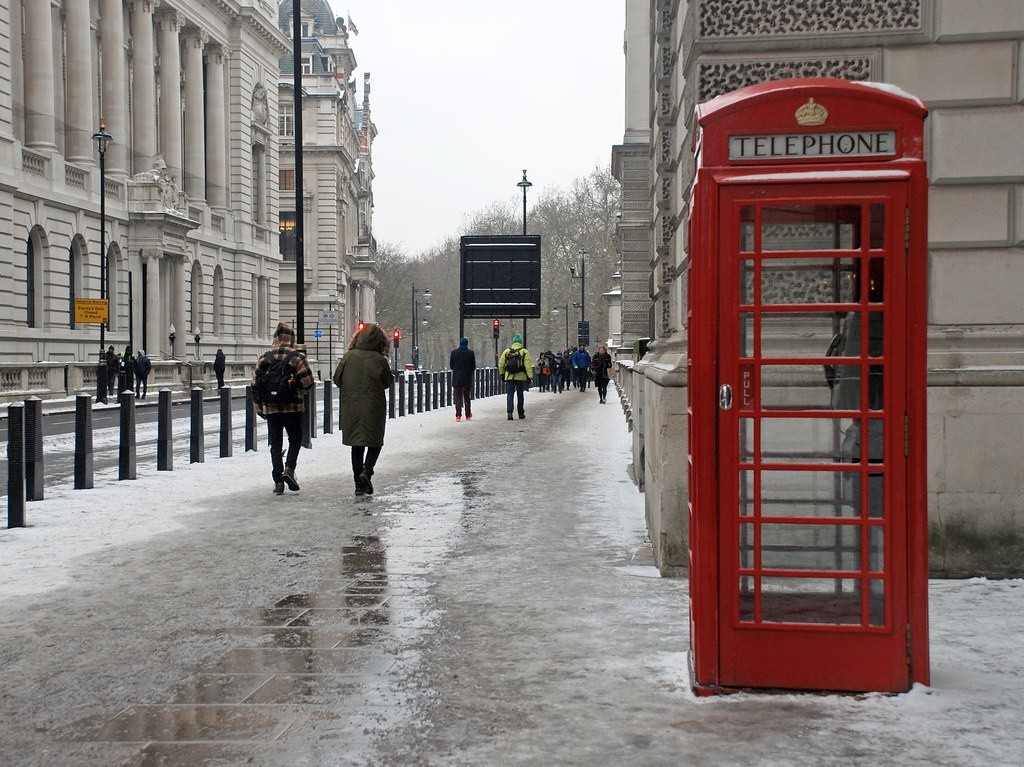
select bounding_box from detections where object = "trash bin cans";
[404,364,414,381]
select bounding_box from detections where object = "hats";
[459,337,468,348]
[513,334,523,343]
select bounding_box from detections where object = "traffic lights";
[359,323,363,330]
[394,331,399,348]
[493,320,499,339]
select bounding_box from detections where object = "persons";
[332,323,392,496]
[214,348,226,390]
[450,336,476,422]
[107,345,152,404]
[249,323,315,496]
[535,344,599,393]
[592,346,612,404]
[498,333,533,420]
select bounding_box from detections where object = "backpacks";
[505,347,526,374]
[257,349,301,407]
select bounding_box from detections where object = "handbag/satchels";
[543,366,550,376]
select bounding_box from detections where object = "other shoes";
[600,398,606,404]
[282,469,300,491]
[456,414,461,421]
[466,413,473,419]
[538,385,590,393]
[135,395,140,399]
[273,483,284,495]
[360,472,373,494]
[141,394,146,399]
[355,485,365,496]
[508,412,513,420]
[519,413,525,419]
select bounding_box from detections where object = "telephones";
[824,311,882,410]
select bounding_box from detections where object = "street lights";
[90,117,114,404]
[550,301,568,349]
[570,245,589,344]
[411,282,432,369]
[516,169,534,350]
